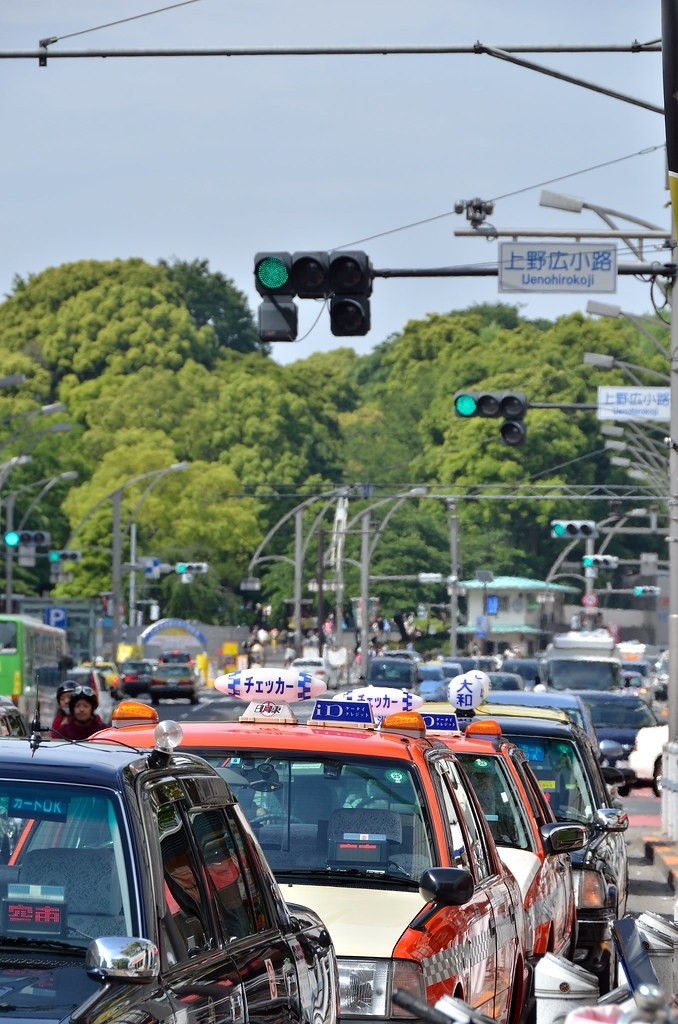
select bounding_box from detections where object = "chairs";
[21,848,127,947]
[251,809,404,873]
[464,773,498,840]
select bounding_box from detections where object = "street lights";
[360,487,427,680]
[539,189,678,834]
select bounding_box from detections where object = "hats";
[69,688,98,713]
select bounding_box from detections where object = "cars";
[337,691,588,1014]
[0,696,31,738]
[67,652,198,705]
[0,738,340,1024]
[87,670,527,1024]
[367,650,670,996]
[289,657,338,690]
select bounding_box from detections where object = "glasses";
[72,686,95,696]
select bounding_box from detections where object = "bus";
[0,615,77,736]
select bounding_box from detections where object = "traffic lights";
[4,469,78,614]
[175,562,209,573]
[4,530,52,547]
[253,250,373,337]
[111,463,188,663]
[48,551,82,562]
[453,391,660,597]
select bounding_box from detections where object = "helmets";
[57,680,81,705]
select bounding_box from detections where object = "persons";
[252,618,333,668]
[50,680,109,740]
[356,616,414,664]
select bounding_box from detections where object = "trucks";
[540,632,621,692]
